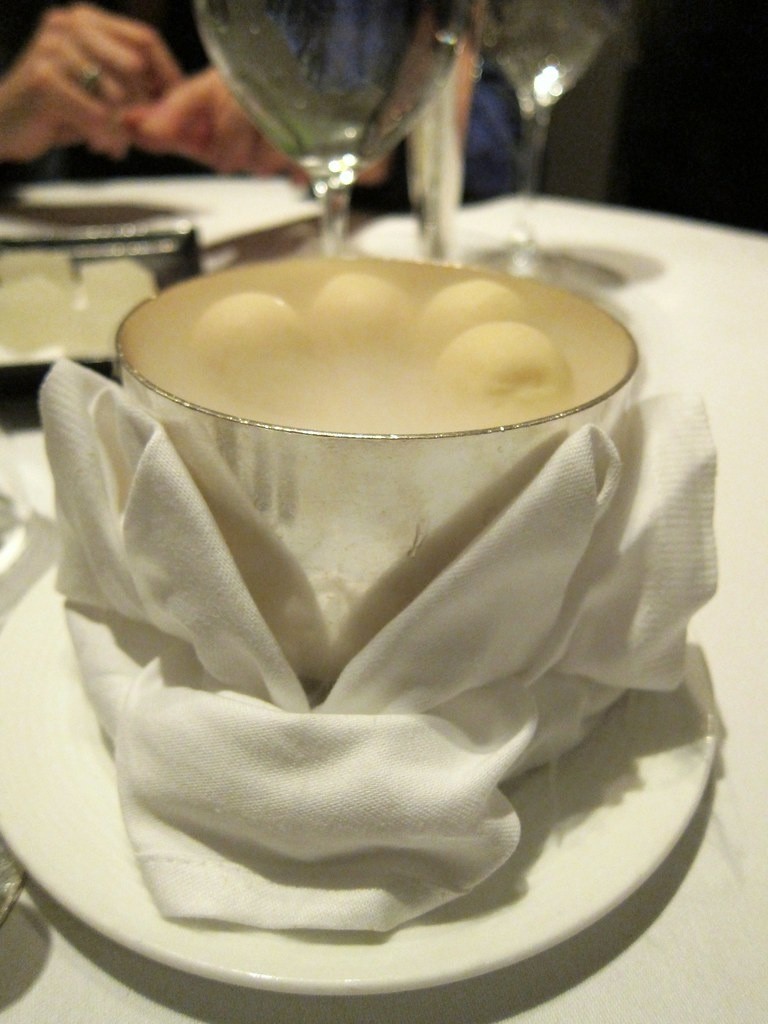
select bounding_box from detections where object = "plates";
[1,174,321,244]
[0,562,714,994]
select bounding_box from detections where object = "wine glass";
[193,1,472,262]
[465,1,628,294]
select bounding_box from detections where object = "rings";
[80,63,102,91]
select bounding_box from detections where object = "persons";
[0,0,523,217]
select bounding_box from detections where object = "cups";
[111,261,639,684]
